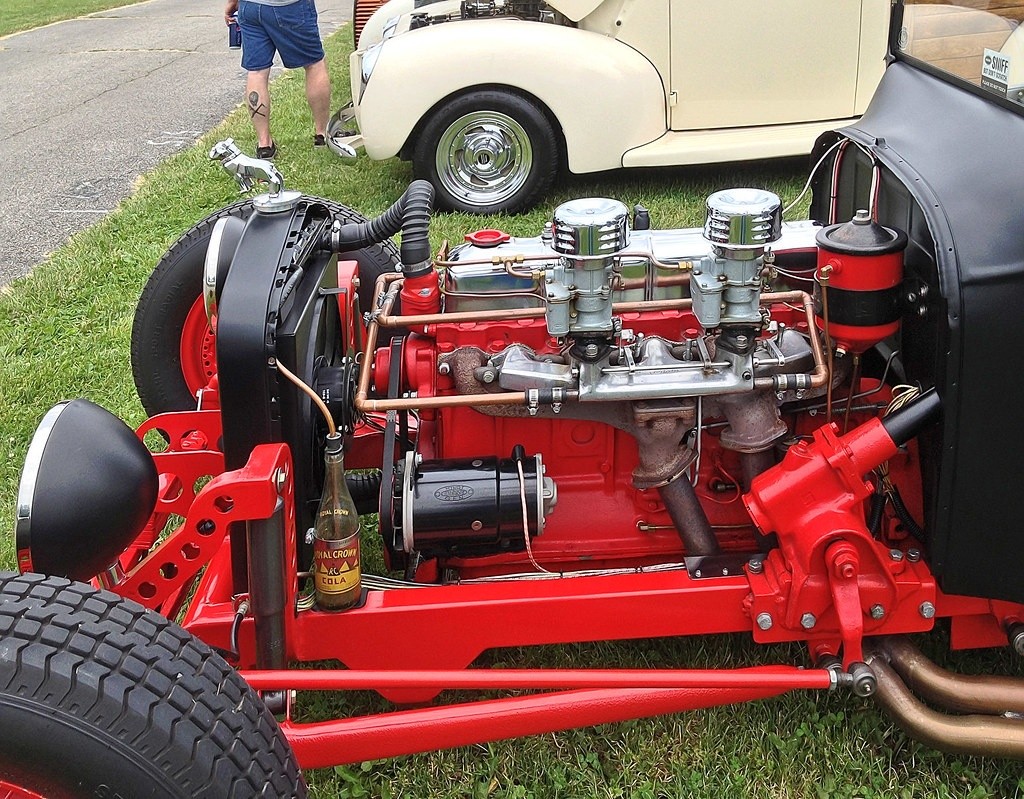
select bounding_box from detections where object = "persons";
[225,0,330,160]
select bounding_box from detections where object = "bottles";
[314,435,360,611]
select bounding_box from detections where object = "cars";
[322,0,1024,218]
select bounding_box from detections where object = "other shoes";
[313,133,329,147]
[254,139,279,159]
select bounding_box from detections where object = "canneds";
[228,15,242,49]
